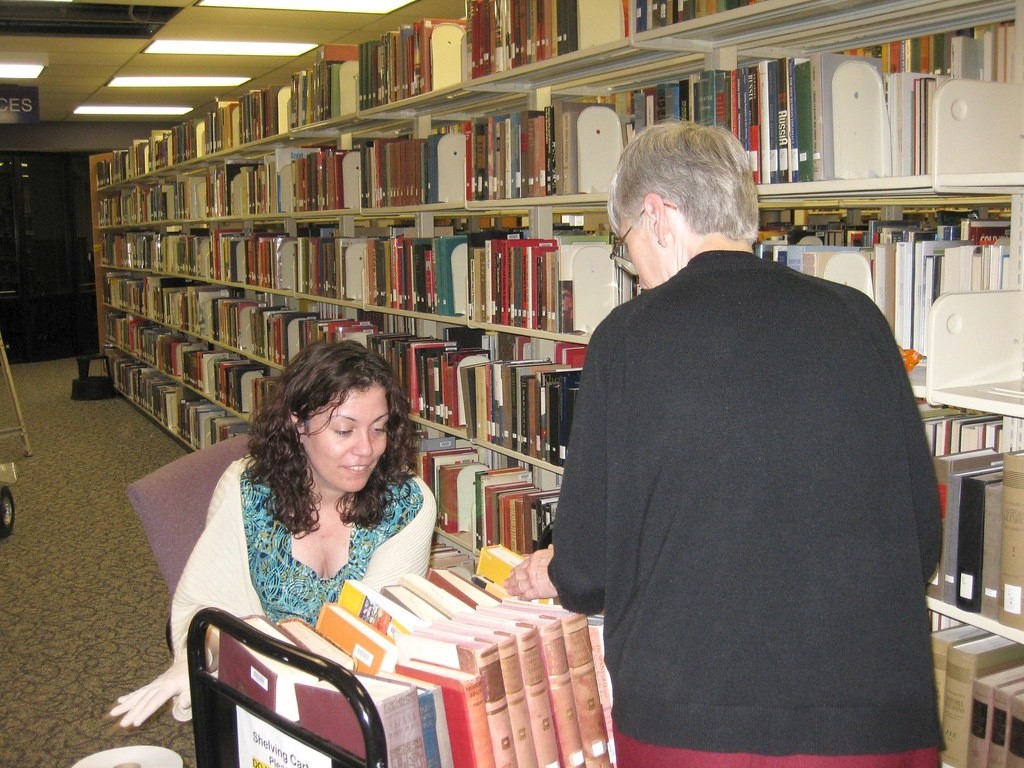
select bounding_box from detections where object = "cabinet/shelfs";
[90,0,1024,768]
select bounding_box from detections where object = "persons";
[501,118,949,768]
[105,338,438,726]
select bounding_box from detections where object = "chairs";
[124,434,256,658]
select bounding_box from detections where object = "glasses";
[609,201,677,276]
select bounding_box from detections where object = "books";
[97,1,757,190]
[926,610,1024,768]
[97,21,1016,230]
[105,270,590,470]
[100,307,562,578]
[232,542,626,768]
[750,209,1013,371]
[909,392,1023,634]
[101,224,651,350]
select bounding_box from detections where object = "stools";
[71,354,115,400]
